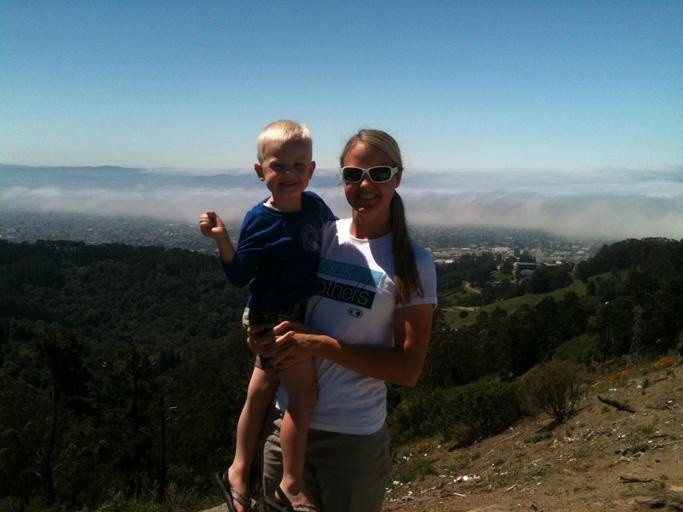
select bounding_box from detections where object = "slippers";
[215,470,252,512]
[264,483,320,512]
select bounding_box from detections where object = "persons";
[247,129,439,512]
[199,121,340,512]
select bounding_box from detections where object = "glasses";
[340,164,399,184]
